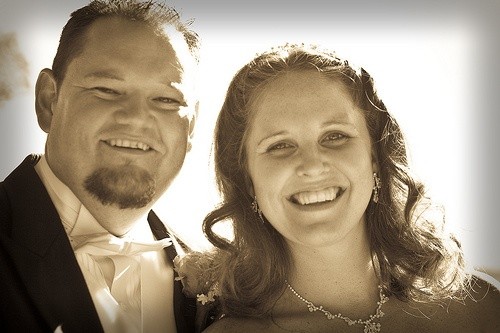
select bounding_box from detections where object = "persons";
[199,39,500,333]
[0,0,226,333]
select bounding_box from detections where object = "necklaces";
[283,279,390,332]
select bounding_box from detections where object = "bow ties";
[74,234,174,258]
